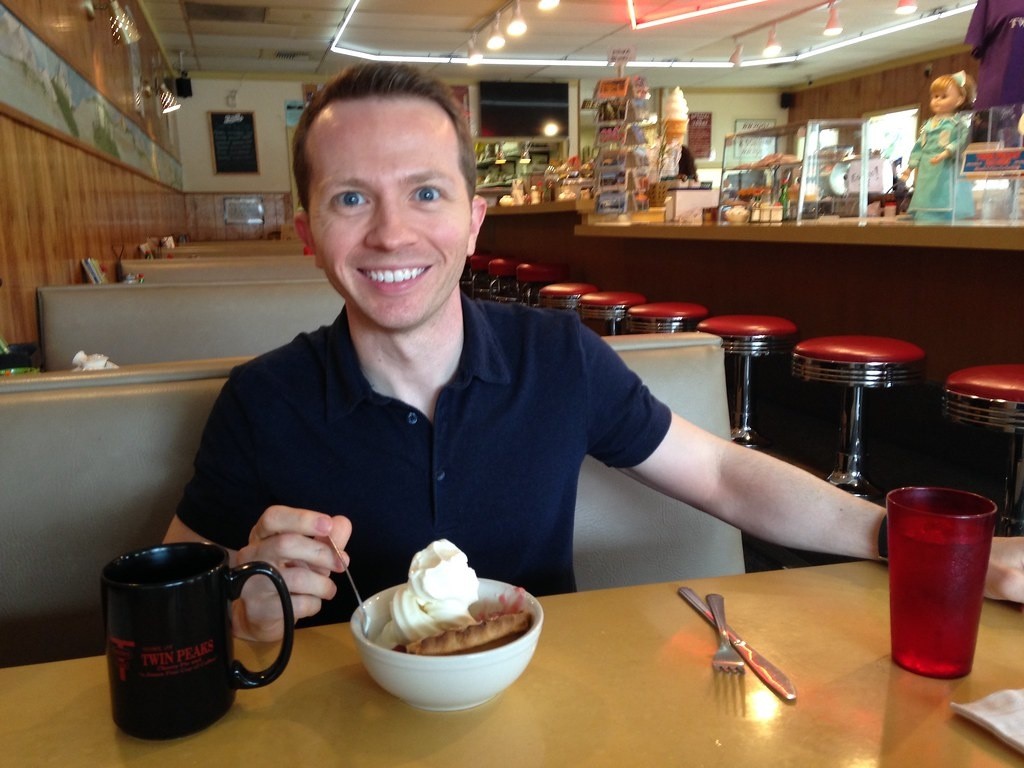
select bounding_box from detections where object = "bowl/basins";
[349,578,543,712]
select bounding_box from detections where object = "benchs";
[1,241,744,668]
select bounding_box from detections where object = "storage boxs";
[849,158,895,195]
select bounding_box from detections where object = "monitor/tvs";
[478,80,570,138]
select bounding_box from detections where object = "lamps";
[83,0,141,46]
[730,37,743,64]
[506,0,527,36]
[896,0,917,15]
[761,22,782,59]
[822,0,843,36]
[486,11,505,50]
[144,80,181,114]
[466,31,484,66]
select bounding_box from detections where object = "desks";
[0,555,1023,768]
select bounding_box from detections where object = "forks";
[703,593,747,676]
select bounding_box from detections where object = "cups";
[886,485,998,679]
[99,539,295,741]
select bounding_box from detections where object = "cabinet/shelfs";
[475,141,531,193]
[719,118,870,222]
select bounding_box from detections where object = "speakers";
[176,78,192,98]
[780,93,791,109]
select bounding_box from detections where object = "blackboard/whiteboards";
[206,110,264,177]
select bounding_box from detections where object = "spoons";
[318,532,374,635]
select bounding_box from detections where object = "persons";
[162,63,1024,643]
[901,70,976,227]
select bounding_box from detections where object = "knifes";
[678,585,797,701]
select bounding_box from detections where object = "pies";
[735,152,801,168]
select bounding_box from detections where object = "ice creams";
[664,85,690,147]
[372,539,531,655]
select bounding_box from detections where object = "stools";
[462,253,1024,541]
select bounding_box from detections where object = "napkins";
[949,689,1024,756]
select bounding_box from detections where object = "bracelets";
[878,512,891,566]
